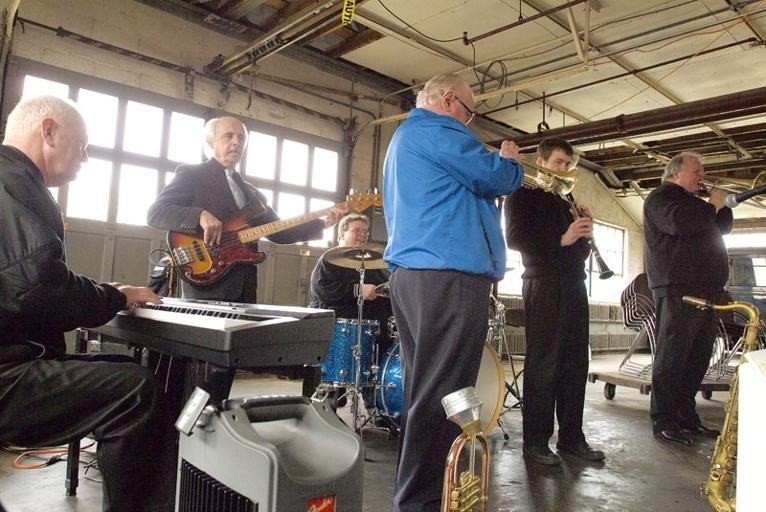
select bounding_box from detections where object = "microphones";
[724,185,766,208]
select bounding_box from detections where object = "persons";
[503,136,606,466]
[307,212,393,409]
[381,73,524,511]
[145,115,351,404]
[643,152,736,448]
[1,94,172,512]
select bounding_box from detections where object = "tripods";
[485,310,509,439]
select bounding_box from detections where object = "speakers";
[363,205,389,243]
[174,397,364,512]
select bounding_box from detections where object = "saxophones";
[682,294,766,512]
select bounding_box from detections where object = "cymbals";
[325,243,387,269]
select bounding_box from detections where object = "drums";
[373,337,505,439]
[322,315,381,390]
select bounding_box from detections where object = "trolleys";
[587,369,735,403]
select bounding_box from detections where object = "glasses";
[454,94,475,126]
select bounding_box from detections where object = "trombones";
[699,172,766,209]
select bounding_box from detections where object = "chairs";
[499,308,526,417]
[618,273,766,381]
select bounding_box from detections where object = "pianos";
[81,294,335,367]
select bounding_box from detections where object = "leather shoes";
[556,438,605,461]
[656,430,693,446]
[693,423,720,436]
[522,444,559,464]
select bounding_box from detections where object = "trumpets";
[483,142,580,195]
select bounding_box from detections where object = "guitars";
[166,187,383,286]
